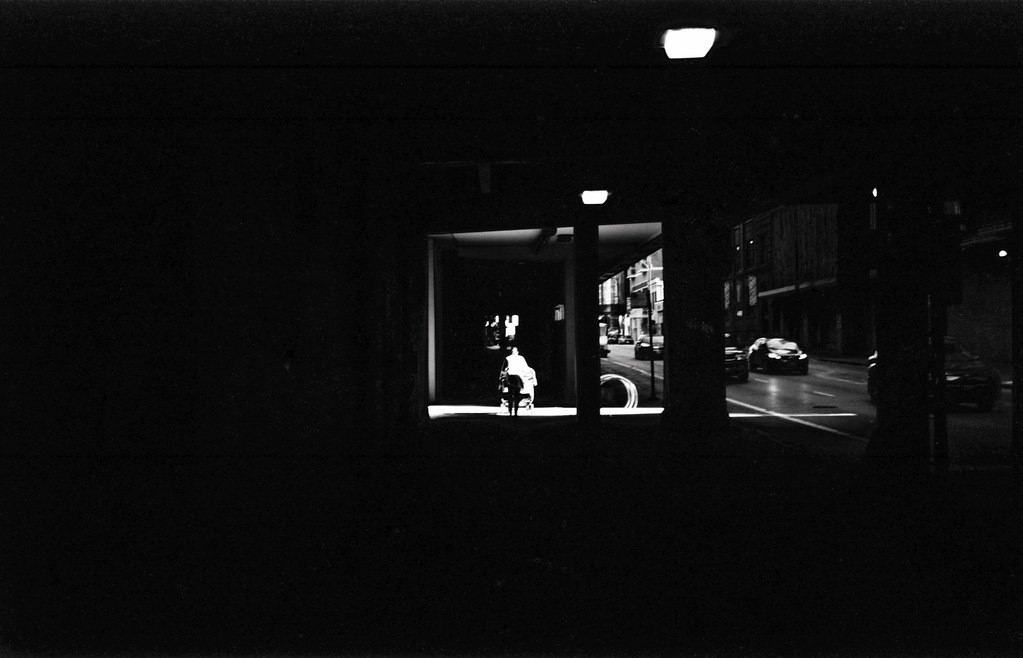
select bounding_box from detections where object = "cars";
[618,335,634,345]
[745,337,810,376]
[635,335,665,361]
[724,346,748,383]
[867,335,1002,412]
